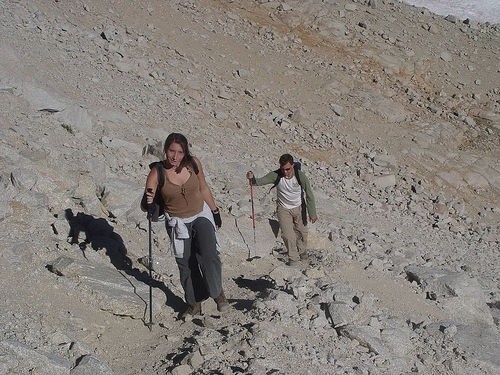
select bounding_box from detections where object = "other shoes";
[289,256,297,266]
[301,252,308,259]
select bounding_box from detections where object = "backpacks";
[274,162,301,185]
[141,157,199,221]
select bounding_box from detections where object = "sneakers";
[178,302,201,319]
[214,288,230,312]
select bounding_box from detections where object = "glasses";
[281,166,291,171]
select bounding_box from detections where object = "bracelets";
[212,207,219,213]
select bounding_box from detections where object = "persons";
[247,153,318,265]
[141,132,233,321]
